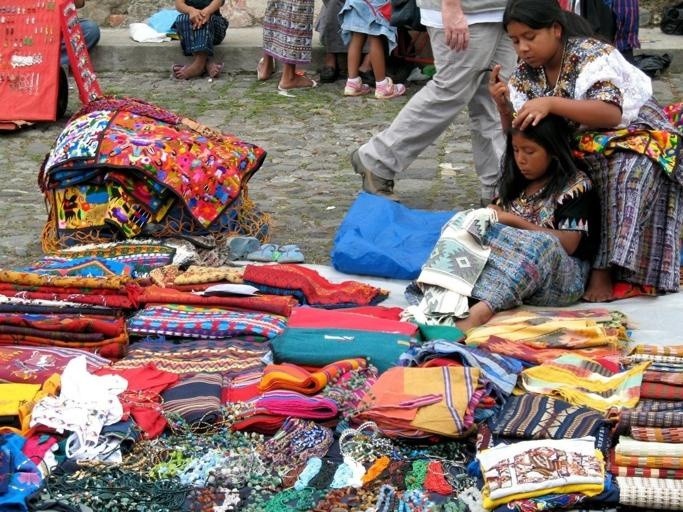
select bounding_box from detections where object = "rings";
[527,113,533,118]
[489,78,494,83]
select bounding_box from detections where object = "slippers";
[170,60,226,82]
[256,55,277,82]
[276,80,318,91]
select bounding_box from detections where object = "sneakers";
[318,64,338,83]
[342,76,371,97]
[374,76,407,99]
[349,147,404,204]
[358,69,391,88]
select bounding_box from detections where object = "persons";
[402,69,603,331]
[314,0,375,88]
[256,0,318,91]
[337,0,406,100]
[60,0,101,64]
[170,0,230,79]
[350,0,520,209]
[487,0,683,303]
